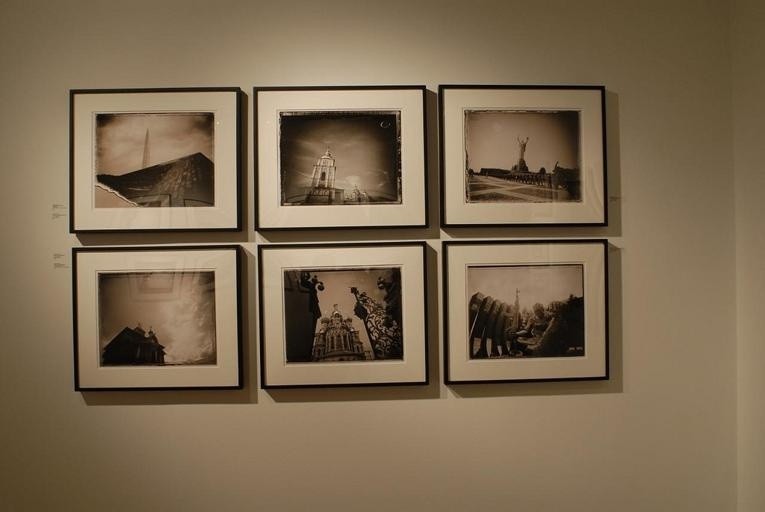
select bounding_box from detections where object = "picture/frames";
[256,241,429,389]
[438,84,608,228]
[441,239,611,385]
[253,86,429,231]
[72,244,245,391]
[70,87,241,234]
